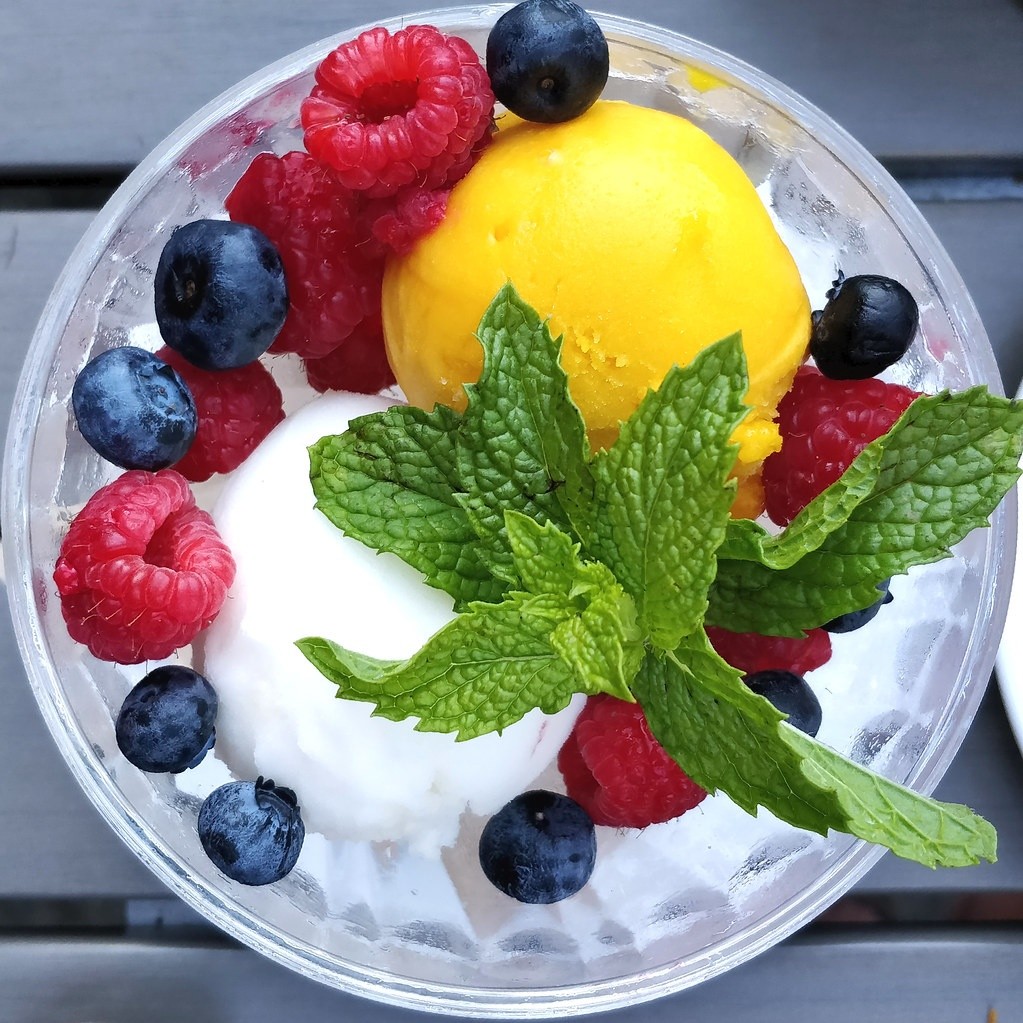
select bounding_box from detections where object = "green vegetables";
[290,282,1022,869]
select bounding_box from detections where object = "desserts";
[191,391,586,850]
[378,98,816,527]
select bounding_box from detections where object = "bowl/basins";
[1,0,1020,1023]
